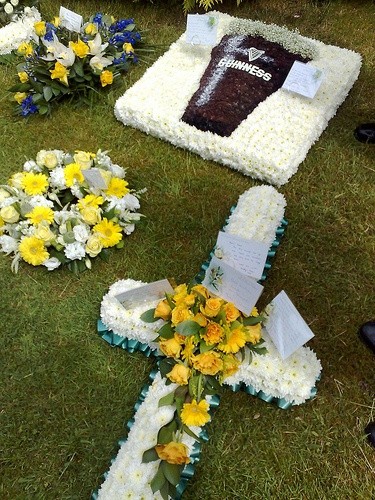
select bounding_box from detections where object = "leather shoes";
[356,121,375,142]
[357,320,375,354]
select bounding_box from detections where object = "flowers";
[113,8,362,188]
[12,10,139,119]
[92,184,322,499]
[0,148,143,274]
[1,1,41,55]
